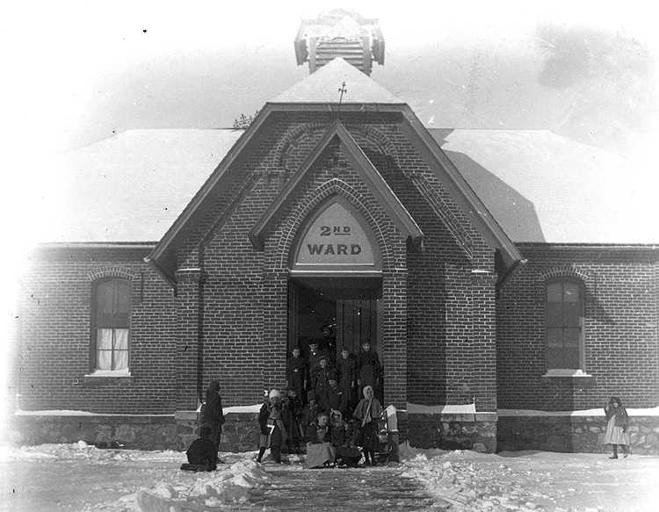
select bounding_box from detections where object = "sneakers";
[609,452,632,459]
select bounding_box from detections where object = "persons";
[603,397,628,459]
[204,378,225,463]
[186,422,217,471]
[256,336,385,469]
